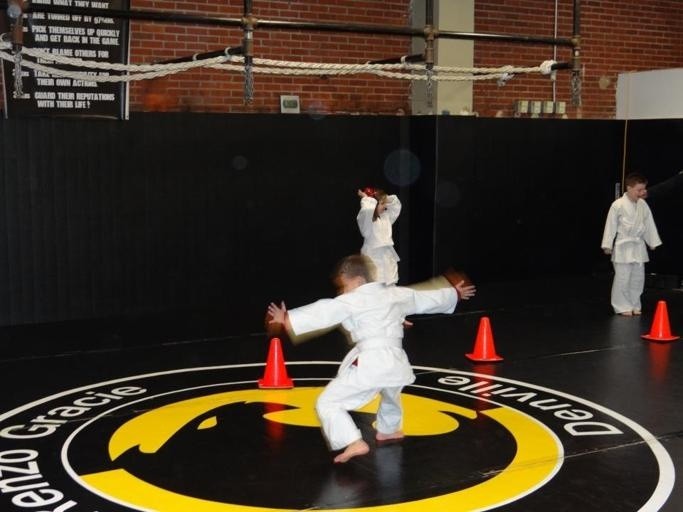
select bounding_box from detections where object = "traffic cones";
[464,316,504,361]
[257,337,294,389]
[640,300,680,342]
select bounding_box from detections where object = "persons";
[357,188,414,327]
[267,254,477,464]
[601,172,663,316]
